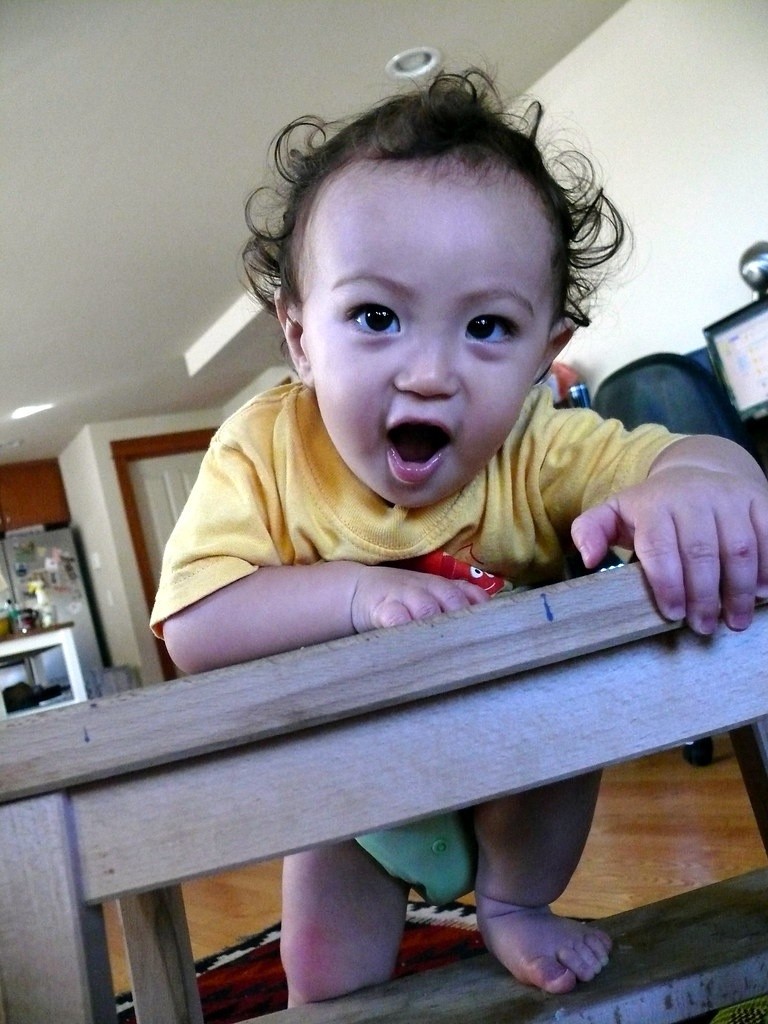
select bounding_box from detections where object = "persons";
[150,68,768,1014]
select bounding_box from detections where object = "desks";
[0,621,88,713]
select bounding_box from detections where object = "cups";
[15,606,39,635]
[38,605,56,629]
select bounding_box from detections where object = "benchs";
[0,541,768,1024]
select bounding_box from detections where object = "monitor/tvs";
[702,293,768,426]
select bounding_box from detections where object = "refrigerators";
[0,522,106,700]
